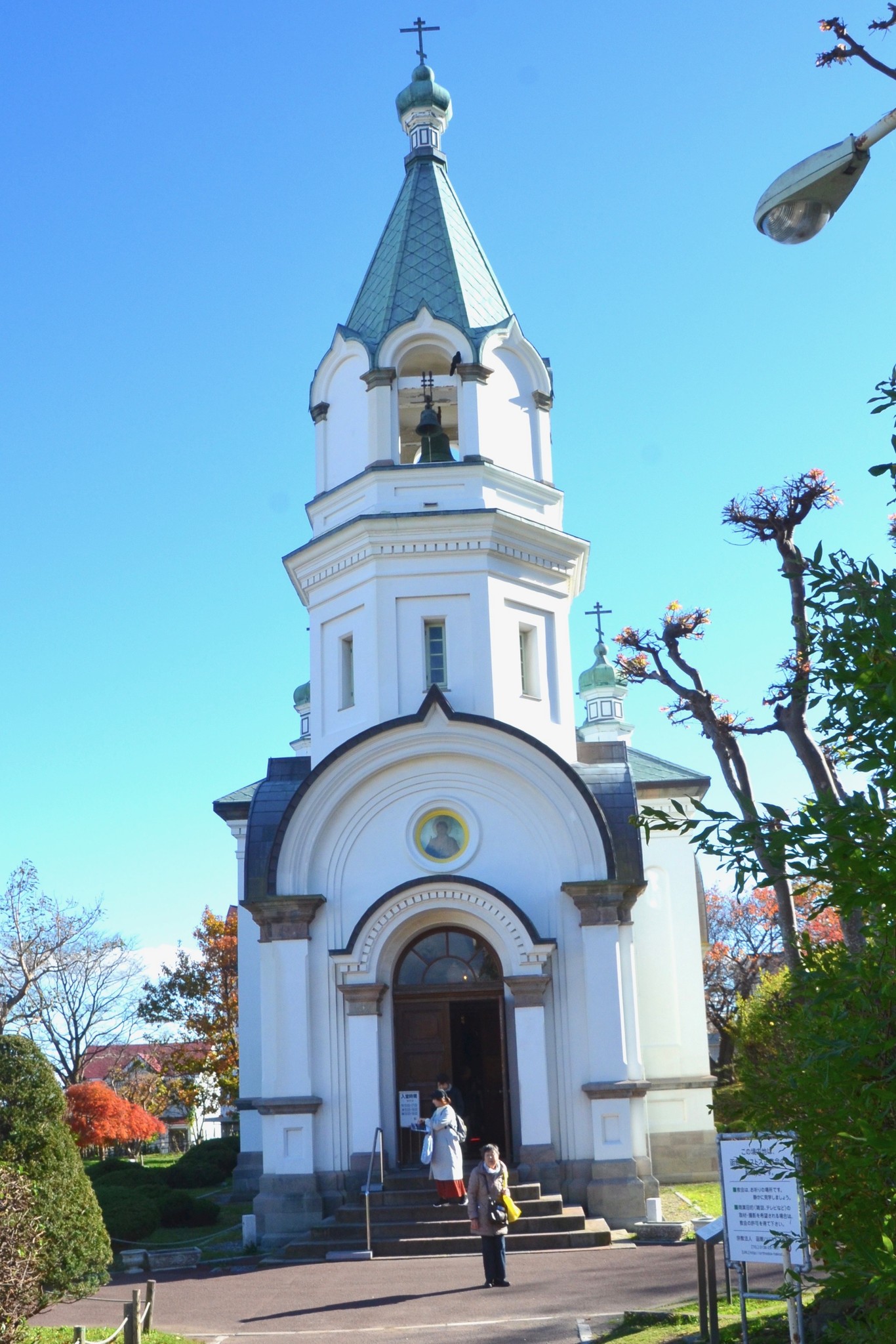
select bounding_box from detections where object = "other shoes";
[495,1279,510,1286]
[484,1279,496,1288]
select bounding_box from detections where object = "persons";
[468,1143,511,1289]
[418,1088,468,1207]
[429,1075,464,1117]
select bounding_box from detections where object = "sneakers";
[458,1195,468,1206]
[433,1198,450,1207]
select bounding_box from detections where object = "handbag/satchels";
[498,1194,522,1223]
[488,1198,509,1226]
[420,1120,434,1165]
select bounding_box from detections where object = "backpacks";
[440,1106,467,1144]
